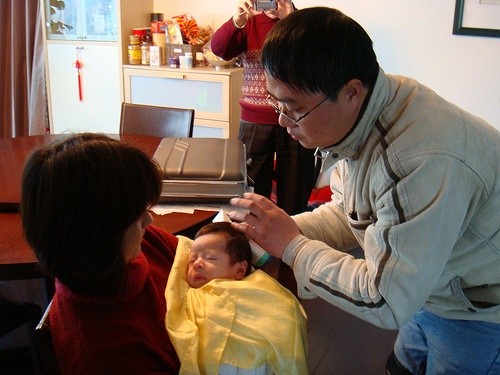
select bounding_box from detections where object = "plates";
[203,55,238,70]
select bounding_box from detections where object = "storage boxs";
[164,42,208,67]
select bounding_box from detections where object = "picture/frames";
[452,0,500,38]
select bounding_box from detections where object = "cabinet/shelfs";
[123,64,244,141]
[40,0,154,134]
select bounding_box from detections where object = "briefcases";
[151,137,249,203]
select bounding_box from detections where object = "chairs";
[117,102,196,140]
[33,297,63,375]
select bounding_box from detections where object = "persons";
[211,0,315,217]
[22,133,180,375]
[187,221,310,375]
[230,7,500,375]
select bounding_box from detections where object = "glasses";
[264,82,346,125]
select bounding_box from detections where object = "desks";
[0,132,222,306]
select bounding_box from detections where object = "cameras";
[254,0,276,11]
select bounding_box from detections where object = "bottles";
[185,52,193,68]
[196,52,204,68]
[150,13,166,65]
